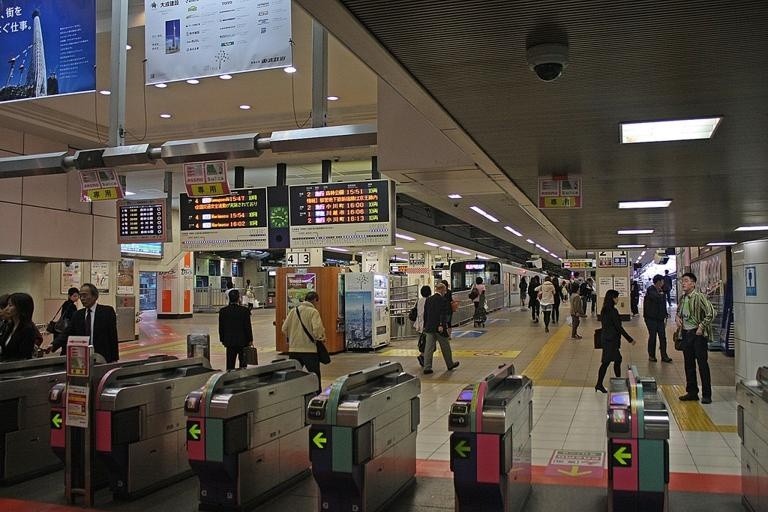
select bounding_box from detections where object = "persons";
[520,275,597,339]
[674,272,716,404]
[642,274,673,362]
[0,283,121,364]
[411,279,461,375]
[630,268,676,317]
[218,290,253,370]
[469,276,487,328]
[243,279,256,315]
[224,281,243,306]
[281,290,326,396]
[595,288,637,394]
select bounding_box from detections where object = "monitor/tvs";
[116,199,166,242]
[121,243,165,259]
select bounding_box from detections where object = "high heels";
[595,384,607,393]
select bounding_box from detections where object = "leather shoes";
[448,362,459,370]
[424,370,433,374]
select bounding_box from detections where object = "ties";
[85,308,92,345]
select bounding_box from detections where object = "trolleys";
[471,307,489,328]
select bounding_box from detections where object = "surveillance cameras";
[65,261,70,267]
[526,45,568,83]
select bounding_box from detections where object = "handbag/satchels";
[673,326,683,350]
[450,301,458,312]
[418,329,436,352]
[54,303,73,333]
[468,284,478,299]
[409,298,419,321]
[241,344,258,365]
[316,340,330,364]
[594,328,620,349]
[537,286,542,299]
[46,321,56,334]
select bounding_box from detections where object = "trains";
[449,258,568,309]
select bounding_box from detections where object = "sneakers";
[662,356,672,362]
[574,333,582,339]
[702,397,712,404]
[678,394,699,401]
[649,354,657,361]
[417,355,424,367]
